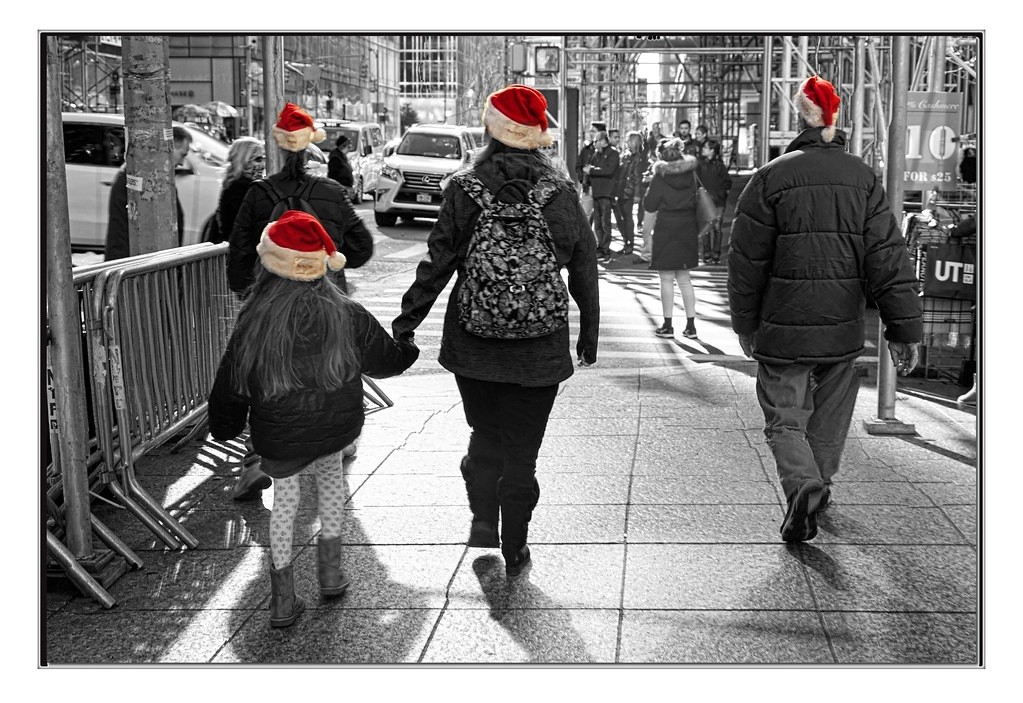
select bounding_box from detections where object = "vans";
[59,112,235,253]
[309,122,387,205]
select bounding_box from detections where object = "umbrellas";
[201,101,239,117]
[173,104,209,117]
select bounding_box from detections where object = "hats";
[795,76,841,143]
[273,102,326,152]
[482,84,554,150]
[257,210,347,280]
[335,135,352,148]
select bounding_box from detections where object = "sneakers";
[231,452,272,501]
[654,327,676,338]
[681,329,698,338]
[340,444,356,459]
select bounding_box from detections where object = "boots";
[957,374,977,404]
[317,533,351,595]
[268,563,306,627]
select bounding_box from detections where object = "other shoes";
[819,489,833,512]
[780,480,829,541]
[617,247,631,255]
[632,256,642,265]
[504,546,530,576]
[466,520,500,548]
[596,250,604,260]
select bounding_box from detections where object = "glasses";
[250,155,268,162]
[592,138,602,144]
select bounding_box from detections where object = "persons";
[225,103,374,502]
[208,211,420,625]
[201,137,264,300]
[643,137,699,338]
[328,135,354,194]
[103,127,192,331]
[540,141,570,177]
[390,84,600,574]
[728,75,922,542]
[574,119,732,263]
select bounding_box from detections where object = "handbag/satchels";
[923,232,978,302]
[692,168,718,238]
[579,183,594,216]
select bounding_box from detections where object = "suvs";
[371,123,478,227]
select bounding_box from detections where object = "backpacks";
[452,169,568,340]
[262,171,320,225]
